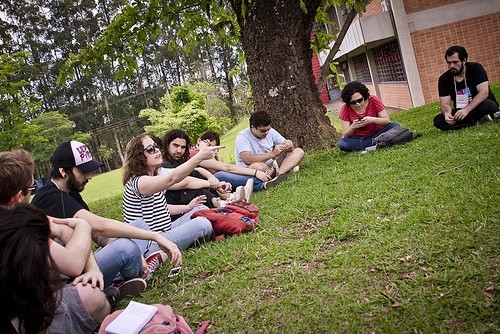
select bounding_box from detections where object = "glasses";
[143,143,160,154]
[16,179,38,193]
[349,96,364,105]
[254,127,271,134]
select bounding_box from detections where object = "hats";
[52,140,105,172]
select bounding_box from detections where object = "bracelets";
[253,170,258,177]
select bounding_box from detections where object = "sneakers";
[227,186,245,205]
[104,287,120,305]
[244,179,254,203]
[105,277,147,297]
[140,251,168,280]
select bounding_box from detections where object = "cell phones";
[168,267,182,277]
[358,116,364,121]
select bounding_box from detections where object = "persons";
[0,149,146,305]
[183,131,288,190]
[0,202,55,334]
[123,134,225,257]
[235,111,304,175]
[433,46,500,130]
[161,129,254,223]
[339,81,400,152]
[30,140,182,296]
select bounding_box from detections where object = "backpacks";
[97,304,210,334]
[376,126,422,150]
[190,199,260,240]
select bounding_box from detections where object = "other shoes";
[479,114,493,124]
[264,173,288,189]
[290,166,299,172]
